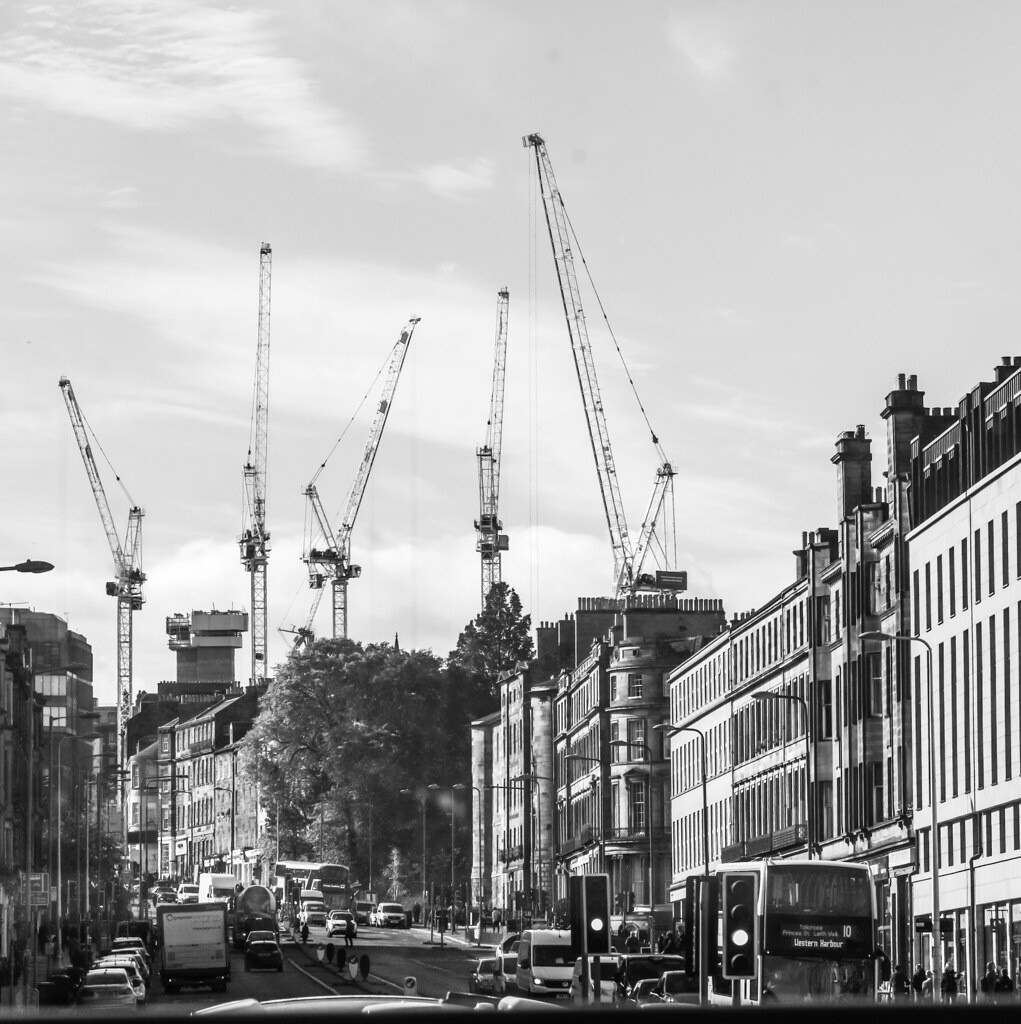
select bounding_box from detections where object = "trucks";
[156,899,232,994]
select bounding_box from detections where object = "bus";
[669,859,892,1008]
[270,859,353,911]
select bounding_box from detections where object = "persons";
[987,961,1018,1005]
[413,900,420,923]
[941,962,962,1004]
[492,907,501,934]
[344,915,354,947]
[627,933,638,953]
[891,965,910,1000]
[661,925,684,956]
[913,964,933,1002]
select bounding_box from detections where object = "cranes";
[57,376,148,758]
[474,283,512,618]
[277,311,422,638]
[520,130,678,598]
[242,244,273,678]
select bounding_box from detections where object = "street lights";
[752,690,812,862]
[510,776,541,917]
[609,740,656,953]
[139,773,189,920]
[428,783,457,935]
[144,778,176,921]
[453,783,483,930]
[75,752,117,940]
[520,774,557,929]
[214,787,234,873]
[857,630,941,1008]
[652,723,710,875]
[47,712,102,931]
[85,764,123,935]
[52,732,104,980]
[400,788,427,928]
[564,754,604,873]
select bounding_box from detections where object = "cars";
[78,869,288,1003]
[287,890,408,938]
[467,911,701,1009]
[74,967,142,1010]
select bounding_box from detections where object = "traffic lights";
[582,873,612,956]
[287,880,295,898]
[627,890,636,913]
[291,887,300,906]
[723,872,757,979]
[285,873,291,894]
[615,890,627,911]
